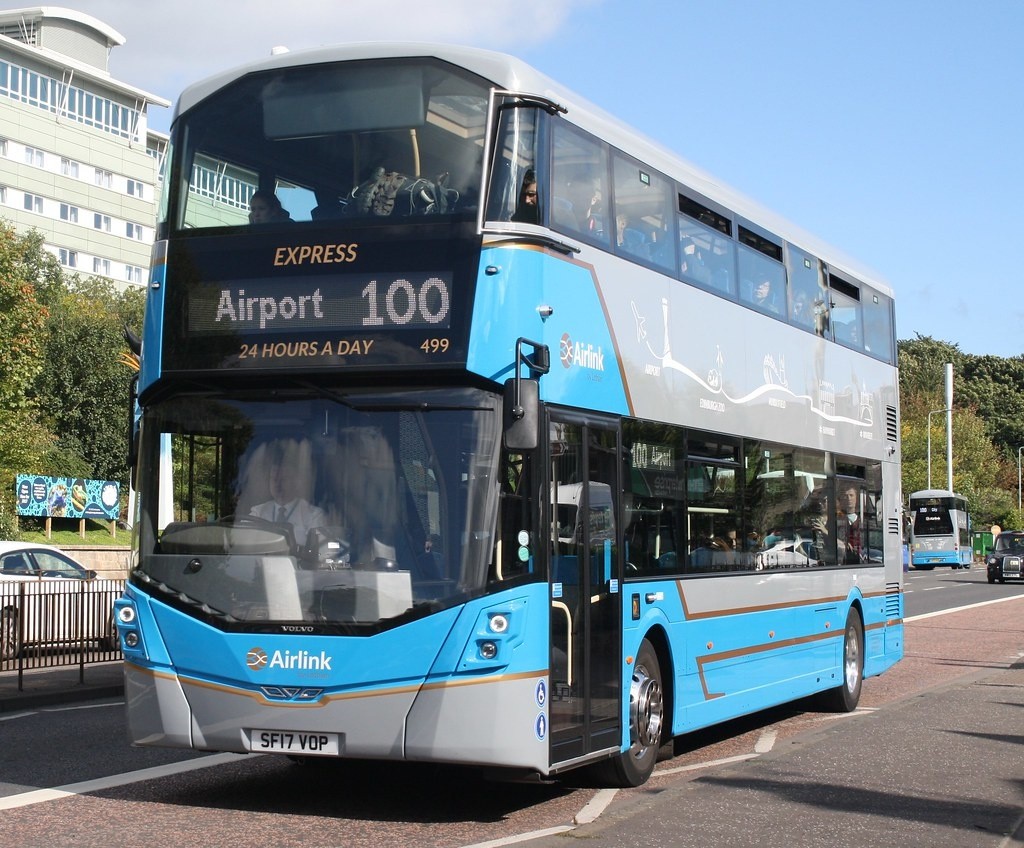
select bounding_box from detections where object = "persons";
[689,481,867,568]
[250,191,296,222]
[519,169,873,352]
[247,461,329,546]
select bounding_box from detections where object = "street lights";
[926,409,955,489]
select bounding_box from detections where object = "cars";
[0,542,125,662]
[986,530,1023,583]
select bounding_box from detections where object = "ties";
[276,507,287,522]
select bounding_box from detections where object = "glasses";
[522,192,536,197]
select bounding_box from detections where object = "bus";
[909,489,973,570]
[111,41,903,790]
